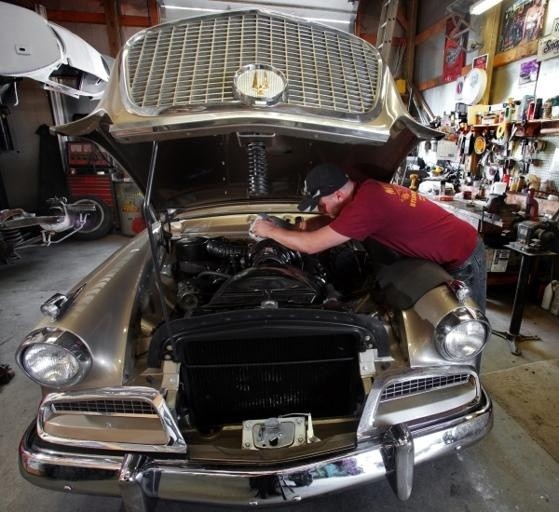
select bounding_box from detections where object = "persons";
[245,161,488,377]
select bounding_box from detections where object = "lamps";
[467,0,507,15]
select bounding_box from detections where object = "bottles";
[427,94,559,203]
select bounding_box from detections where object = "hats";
[297,166,349,212]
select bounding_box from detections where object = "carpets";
[480,354,558,464]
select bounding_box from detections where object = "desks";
[487,242,558,355]
[432,190,525,313]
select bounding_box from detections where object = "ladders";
[375,0,400,63]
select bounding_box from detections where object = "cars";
[15,9,494,511]
